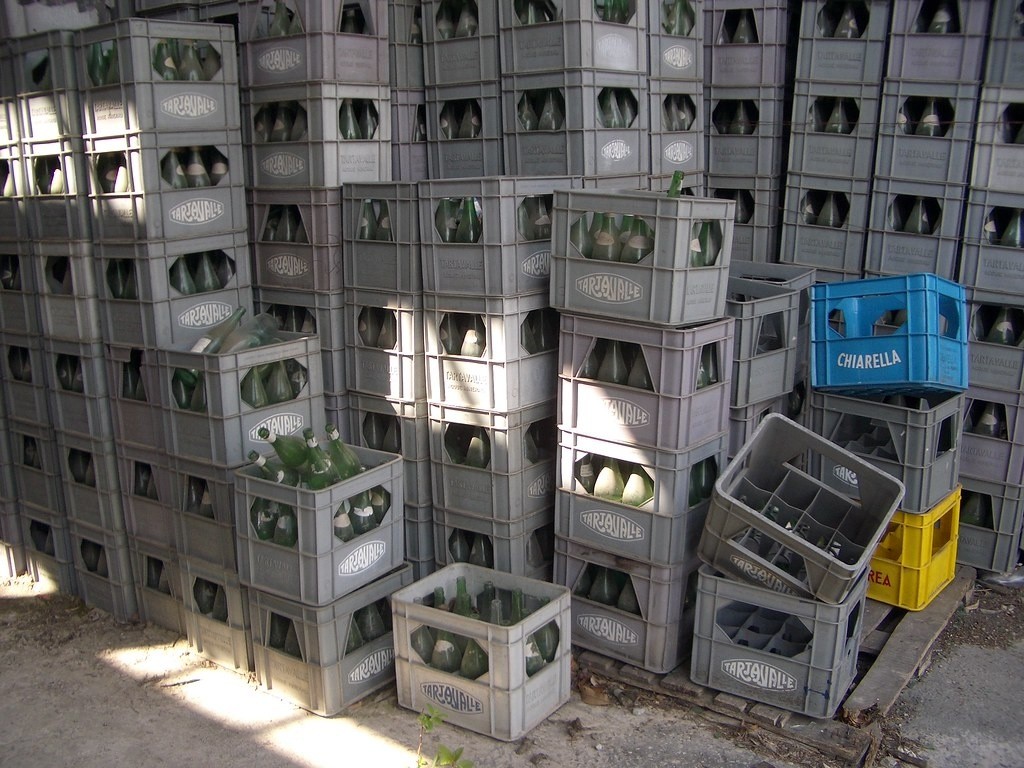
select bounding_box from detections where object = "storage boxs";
[0,0,1024,743]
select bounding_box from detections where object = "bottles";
[0,0,1024,679]
